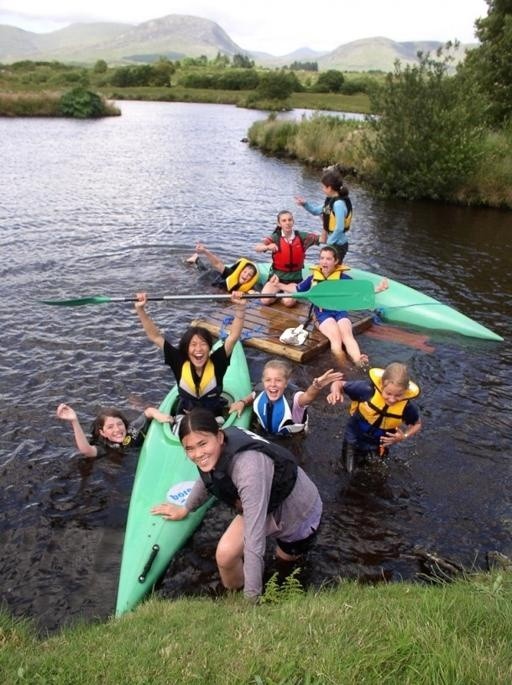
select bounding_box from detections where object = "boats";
[220,262,505,342]
[113,334,255,627]
[190,296,375,363]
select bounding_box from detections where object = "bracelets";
[235,312,246,320]
[403,433,409,440]
[311,378,324,389]
[238,400,247,408]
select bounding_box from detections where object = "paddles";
[278,316,314,347]
[37,280,376,312]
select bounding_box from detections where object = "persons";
[55,401,176,460]
[132,289,249,435]
[268,246,390,372]
[325,361,424,474]
[150,408,324,602]
[227,357,345,441]
[184,240,260,305]
[293,170,353,265]
[255,208,325,309]
